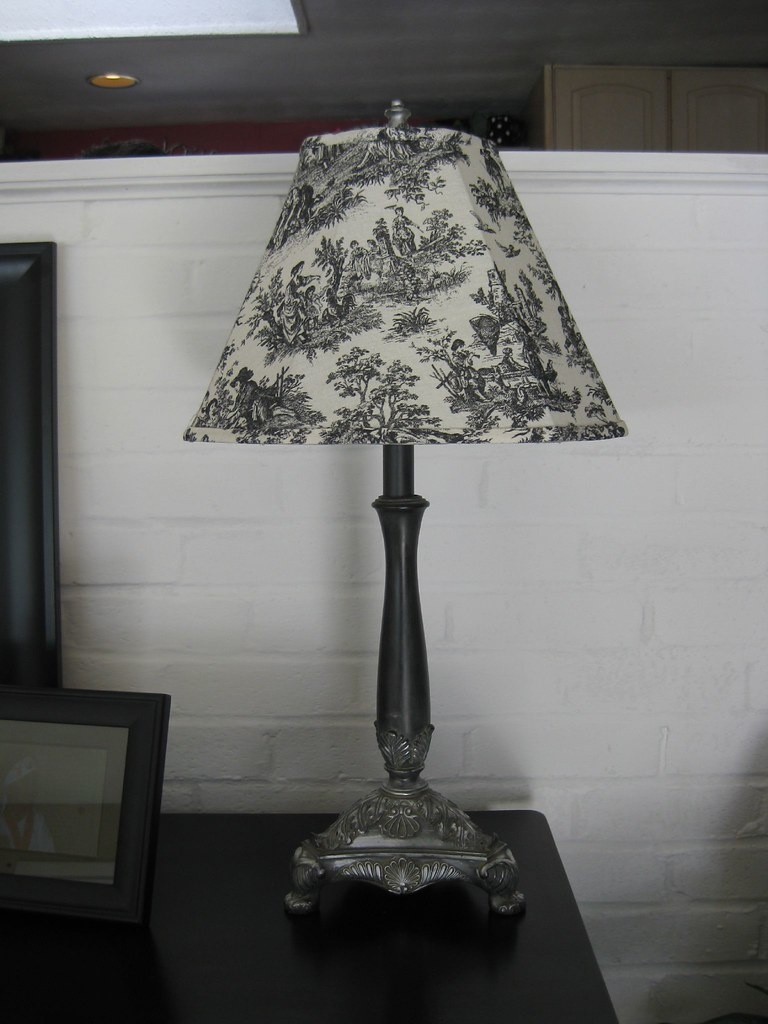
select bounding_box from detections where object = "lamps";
[181,100,628,922]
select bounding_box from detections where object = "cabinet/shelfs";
[523,62,768,152]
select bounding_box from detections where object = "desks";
[0,811,621,1024]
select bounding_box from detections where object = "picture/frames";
[0,243,62,686]
[0,683,172,930]
